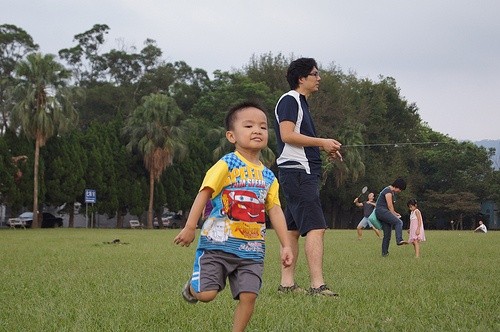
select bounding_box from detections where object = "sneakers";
[308,285,339,297]
[182,275,198,304]
[278,283,308,294]
[397,240,409,245]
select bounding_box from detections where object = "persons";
[355,192,382,241]
[474,220,487,233]
[407,199,426,257]
[173,100,294,332]
[274,58,342,297]
[375,179,409,257]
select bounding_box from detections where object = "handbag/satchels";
[368,207,382,230]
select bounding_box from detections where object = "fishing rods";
[319,139,461,151]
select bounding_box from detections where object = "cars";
[153,211,187,229]
[8,212,63,228]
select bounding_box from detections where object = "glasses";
[307,72,320,77]
